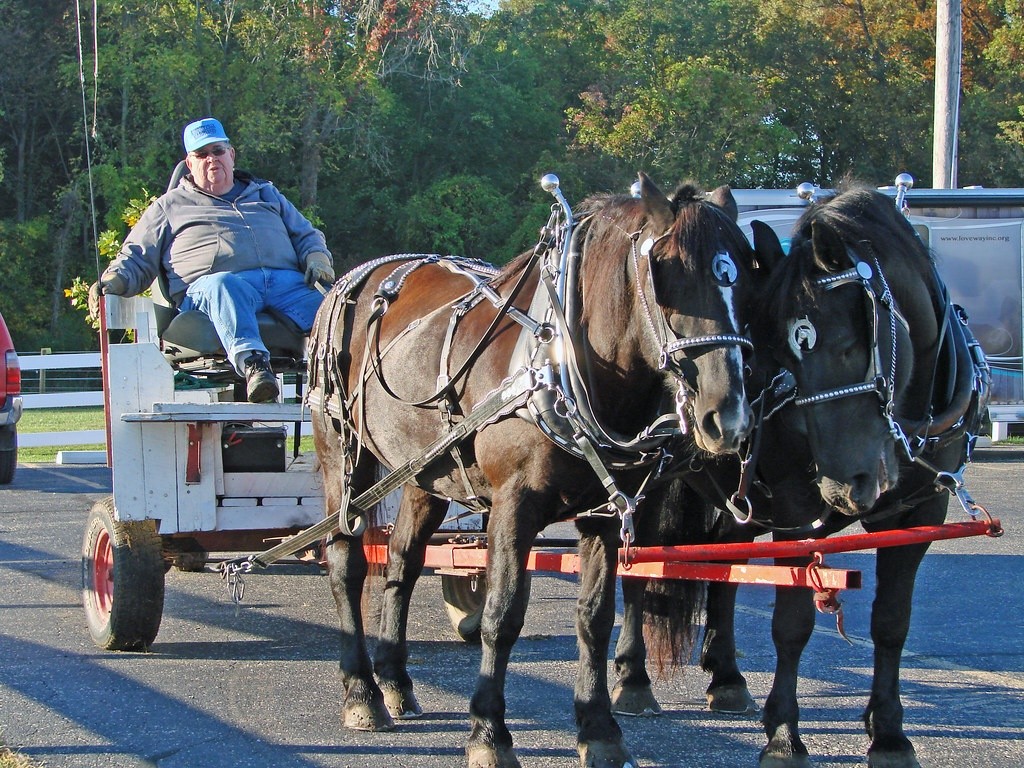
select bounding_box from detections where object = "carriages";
[80,171,1001,768]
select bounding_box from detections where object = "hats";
[184,118,229,154]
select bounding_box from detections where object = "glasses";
[190,145,230,159]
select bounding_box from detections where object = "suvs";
[0,313,23,486]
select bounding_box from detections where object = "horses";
[611,184,995,768]
[302,173,757,768]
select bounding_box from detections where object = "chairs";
[152,268,304,355]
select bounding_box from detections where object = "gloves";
[304,252,335,289]
[87,272,129,322]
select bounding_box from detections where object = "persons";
[89,118,336,403]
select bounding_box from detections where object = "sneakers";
[244,351,280,403]
